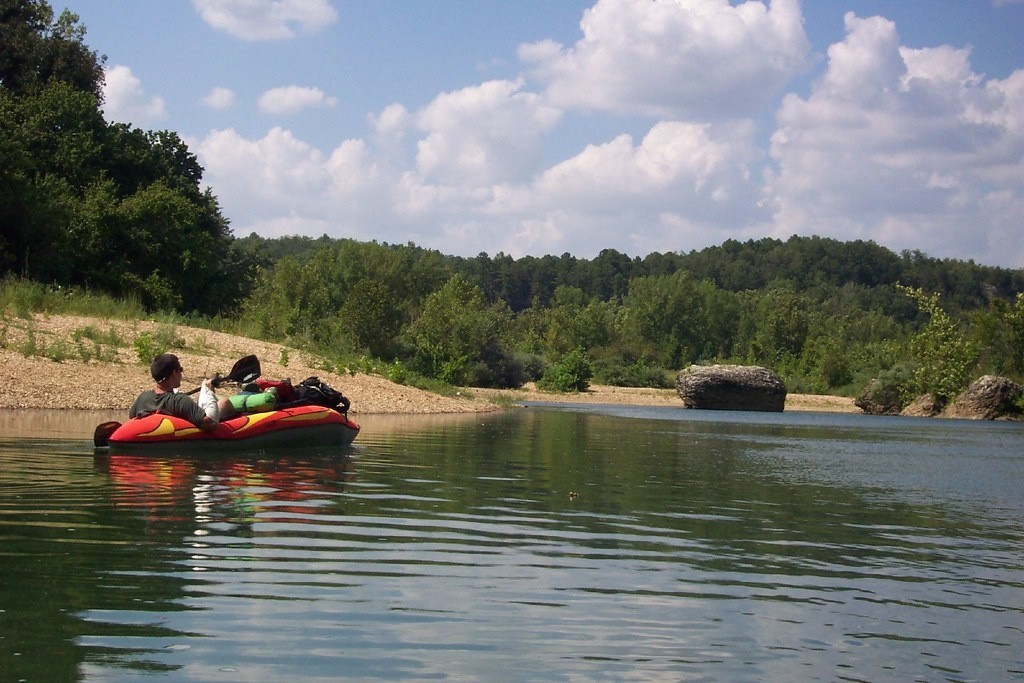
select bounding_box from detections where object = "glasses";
[173,367,183,372]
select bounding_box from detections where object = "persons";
[129,354,235,428]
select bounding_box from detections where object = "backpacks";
[296,377,350,413]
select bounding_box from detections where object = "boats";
[108,405,360,455]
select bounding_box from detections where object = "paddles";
[94,354,261,446]
